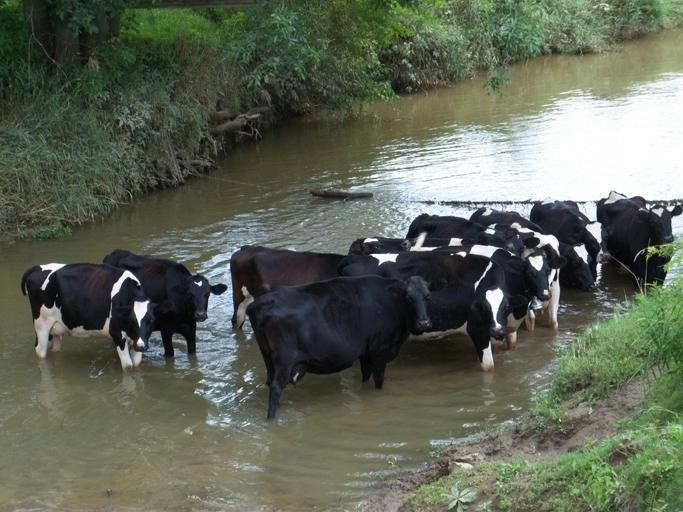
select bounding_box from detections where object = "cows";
[20,261,174,373]
[103,248,228,358]
[229,197,598,420]
[594,190,683,291]
